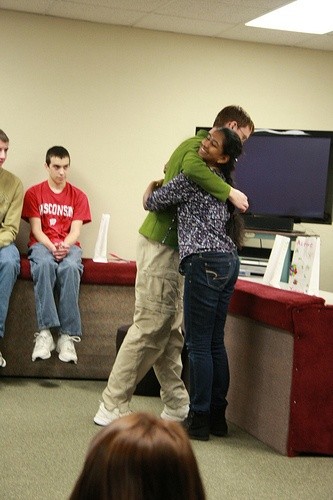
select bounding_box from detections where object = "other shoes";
[0,352,6,367]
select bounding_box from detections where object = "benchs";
[0,253,137,380]
[223,278,333,458]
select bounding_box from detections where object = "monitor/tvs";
[193,126,333,233]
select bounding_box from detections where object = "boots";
[180,409,210,441]
[209,399,229,437]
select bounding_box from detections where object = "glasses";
[229,122,247,143]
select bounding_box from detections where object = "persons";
[70,412,205,500]
[21,146,92,365]
[143,127,242,441]
[0,128,24,367]
[95,106,255,428]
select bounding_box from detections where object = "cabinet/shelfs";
[240,223,320,279]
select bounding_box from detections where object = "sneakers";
[56,334,81,364]
[32,333,55,362]
[161,404,190,422]
[93,400,132,426]
